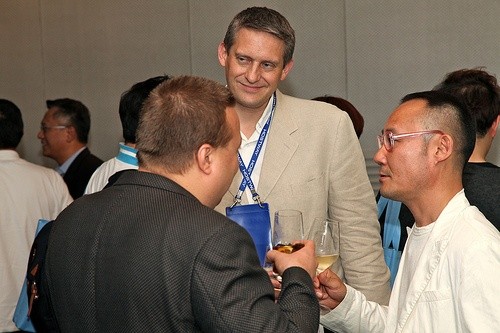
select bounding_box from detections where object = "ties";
[57,169,62,175]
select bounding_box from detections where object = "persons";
[0,98,73,333]
[85,75,171,196]
[313,90,500,333]
[28,75,320,333]
[37,99,104,200]
[214,7,392,306]
[375,67,500,288]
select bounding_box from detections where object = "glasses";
[376,128,444,152]
[40,123,70,133]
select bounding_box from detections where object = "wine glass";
[307,217,340,317]
[271,210,305,291]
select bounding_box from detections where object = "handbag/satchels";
[375,196,403,289]
[12,219,49,332]
